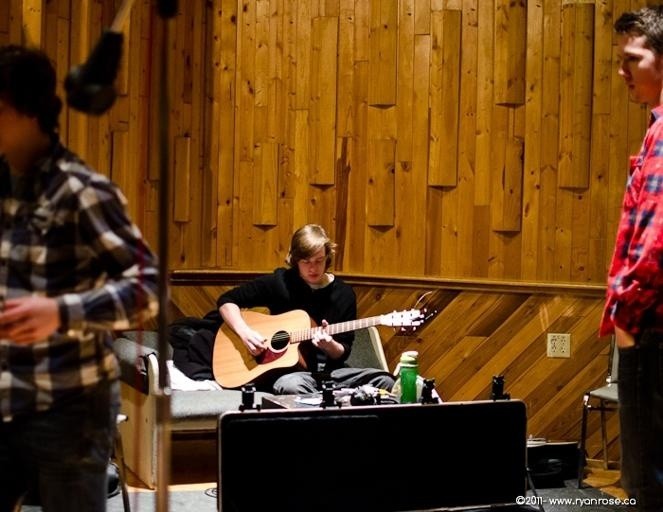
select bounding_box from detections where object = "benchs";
[122,327,391,435]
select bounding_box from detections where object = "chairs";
[12,413,131,512]
[578,330,620,491]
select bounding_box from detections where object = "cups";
[400,351,419,405]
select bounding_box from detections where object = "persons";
[0,43,162,512]
[217,222,395,394]
[594,5,662,512]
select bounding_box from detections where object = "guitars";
[212,307,437,391]
